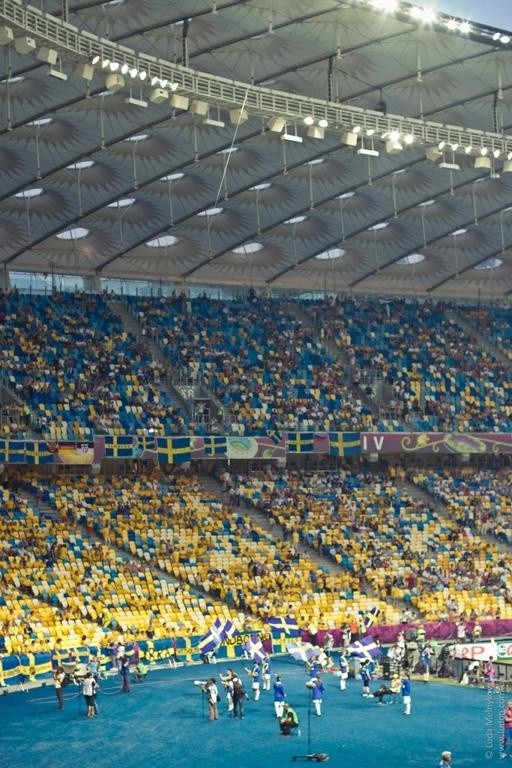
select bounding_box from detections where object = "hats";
[391,673,398,678]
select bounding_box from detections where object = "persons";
[310,674,325,715]
[401,673,411,714]
[83,672,94,716]
[232,677,248,717]
[272,675,287,717]
[500,700,512,757]
[90,676,98,713]
[279,703,299,735]
[201,678,218,720]
[438,750,452,767]
[220,668,235,710]
[53,667,65,711]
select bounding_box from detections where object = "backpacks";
[233,680,243,698]
[57,672,70,688]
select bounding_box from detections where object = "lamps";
[1,13,511,178]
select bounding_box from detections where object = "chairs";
[0,292,512,657]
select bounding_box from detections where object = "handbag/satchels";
[92,687,97,700]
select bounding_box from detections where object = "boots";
[228,702,234,711]
[262,680,270,691]
[421,672,430,681]
[403,703,410,715]
[254,688,260,701]
[314,701,323,716]
[340,680,348,691]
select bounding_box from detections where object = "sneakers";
[234,715,245,720]
[362,693,374,698]
[53,704,100,722]
